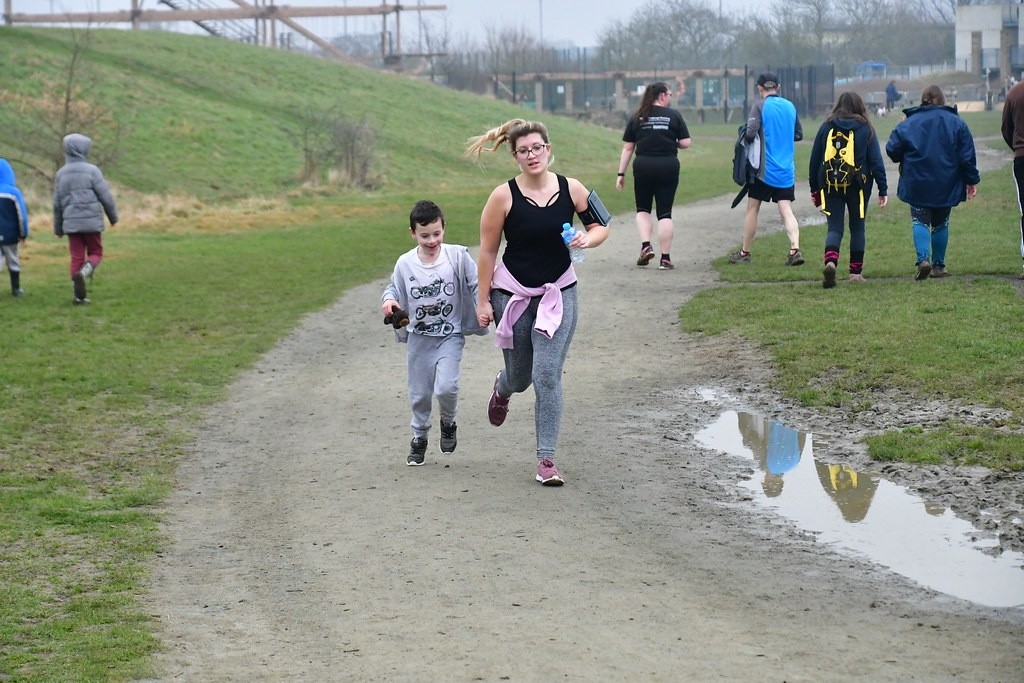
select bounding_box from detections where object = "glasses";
[666,92,672,97]
[515,143,547,159]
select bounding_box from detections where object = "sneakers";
[786,250,806,266]
[487,370,511,427]
[659,258,674,270]
[536,457,565,486]
[406,437,428,465]
[637,245,655,266]
[439,420,458,455]
[823,262,836,289]
[929,265,947,278]
[728,249,750,262]
[914,260,931,281]
[848,273,867,282]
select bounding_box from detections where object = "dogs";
[877,107,886,117]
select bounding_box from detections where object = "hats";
[754,72,779,91]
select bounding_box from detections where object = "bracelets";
[618,173,624,176]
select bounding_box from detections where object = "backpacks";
[819,127,872,218]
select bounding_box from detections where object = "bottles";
[561,223,587,265]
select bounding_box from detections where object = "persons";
[615,82,690,270]
[728,72,805,267]
[0,158,30,297]
[53,134,118,305]
[1001,81,1024,267]
[884,84,980,282]
[886,80,898,112]
[381,199,490,465]
[464,118,610,487]
[808,91,889,289]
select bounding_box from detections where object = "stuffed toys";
[384,306,410,329]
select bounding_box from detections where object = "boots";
[10,270,23,297]
[72,260,95,301]
[73,284,91,305]
